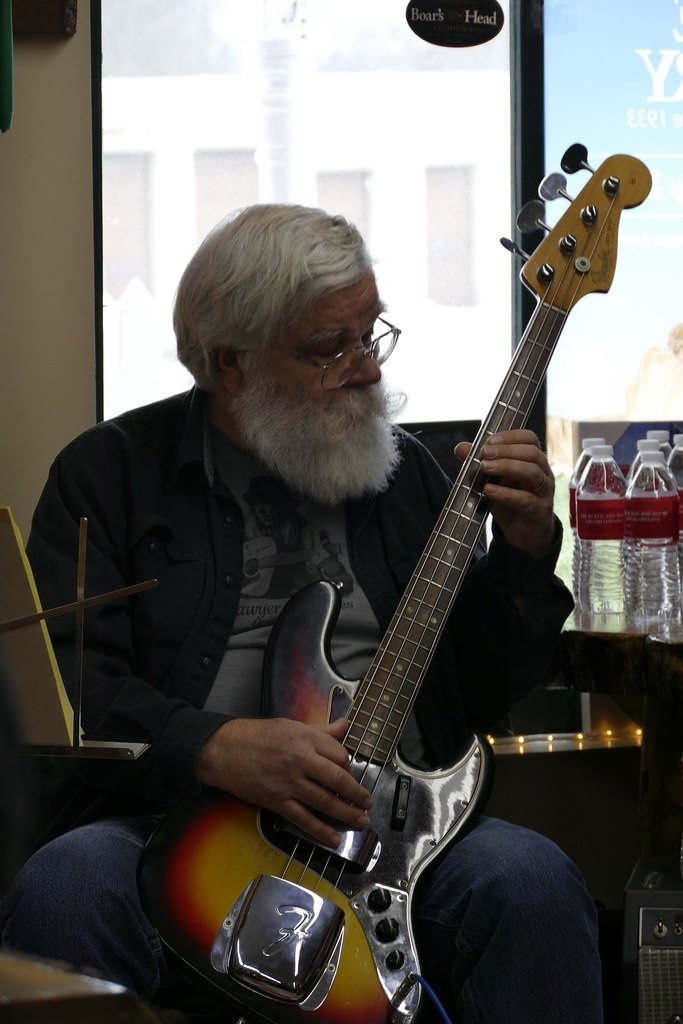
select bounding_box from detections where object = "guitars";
[137,142,651,1023]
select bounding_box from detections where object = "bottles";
[567,437,605,601]
[575,445,626,614]
[625,428,683,623]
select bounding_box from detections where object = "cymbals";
[1,956,127,1004]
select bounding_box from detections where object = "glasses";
[270,317,400,389]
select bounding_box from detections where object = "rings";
[532,474,546,491]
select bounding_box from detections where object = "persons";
[0,204,601,1024]
